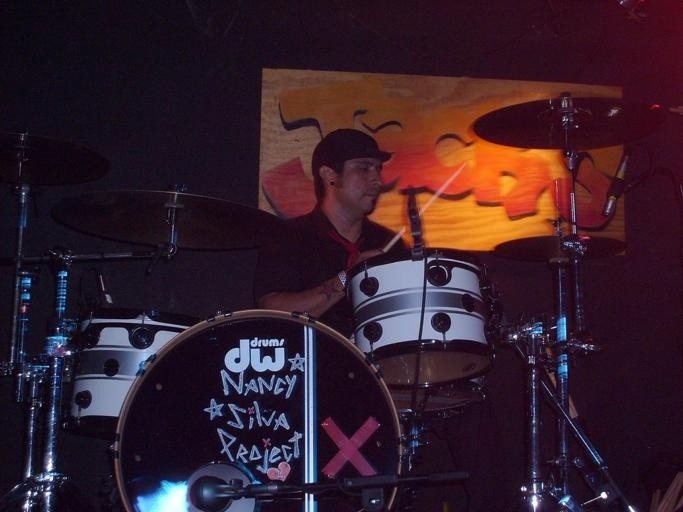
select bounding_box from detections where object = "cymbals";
[50,189,283,251]
[491,235,624,268]
[0,133,107,186]
[473,96,659,149]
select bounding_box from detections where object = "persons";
[249,127,528,512]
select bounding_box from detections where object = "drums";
[73,307,202,452]
[348,252,496,418]
[115,309,401,512]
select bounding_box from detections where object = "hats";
[312,128,391,175]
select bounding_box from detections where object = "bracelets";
[337,267,348,287]
